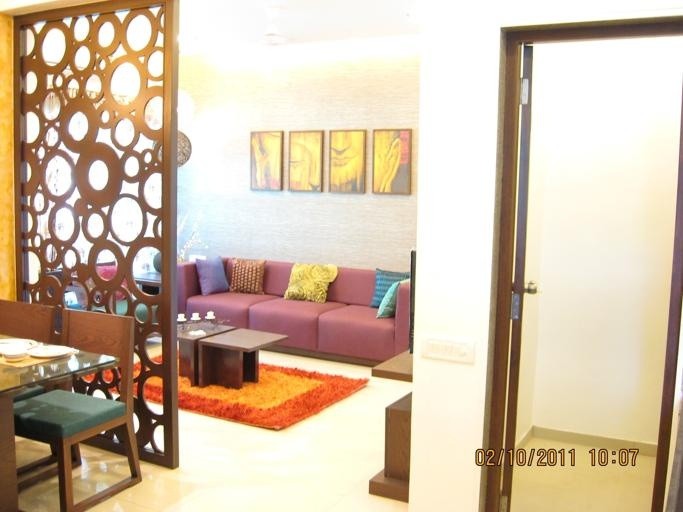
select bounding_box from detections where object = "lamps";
[151,126,192,169]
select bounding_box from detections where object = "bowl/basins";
[3,346,28,361]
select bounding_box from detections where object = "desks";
[0,333,120,512]
[174,318,294,388]
[133,271,165,294]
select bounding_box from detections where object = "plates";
[0,338,36,355]
[28,345,73,358]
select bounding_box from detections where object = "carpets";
[78,346,372,432]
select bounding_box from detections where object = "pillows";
[369,266,409,309]
[193,256,230,295]
[282,260,338,305]
[90,265,132,304]
[227,257,267,295]
[375,279,399,318]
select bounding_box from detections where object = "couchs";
[174,252,412,369]
[38,260,159,336]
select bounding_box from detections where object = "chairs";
[11,300,140,512]
[0,301,82,493]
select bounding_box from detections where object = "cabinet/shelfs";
[368,339,411,501]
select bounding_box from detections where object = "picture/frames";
[326,128,368,197]
[286,128,326,194]
[370,128,414,195]
[246,130,285,193]
